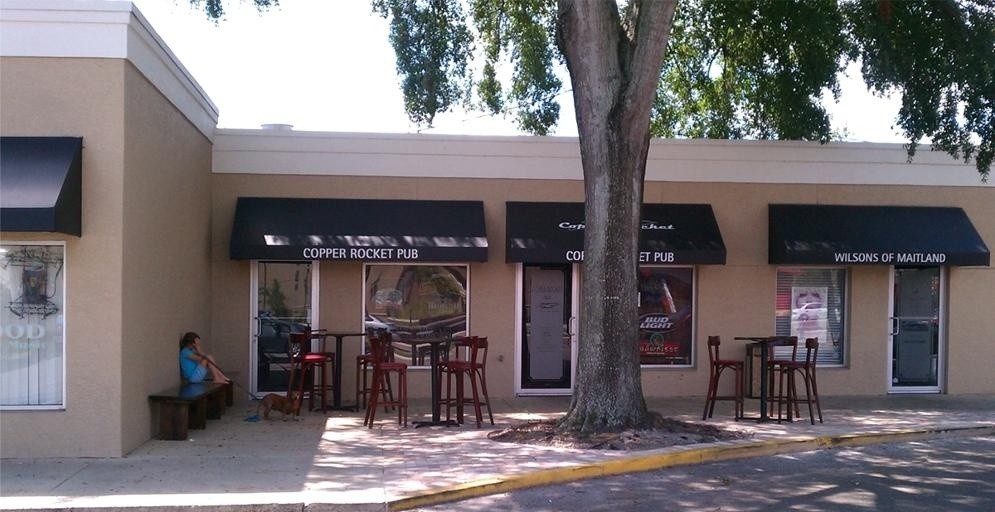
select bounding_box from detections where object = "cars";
[258,309,404,378]
[792,301,838,321]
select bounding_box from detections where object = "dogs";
[256,388,302,421]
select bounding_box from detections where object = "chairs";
[287,328,496,431]
[699,333,825,426]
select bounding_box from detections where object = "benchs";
[148,379,234,442]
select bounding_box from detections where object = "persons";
[791,286,827,344]
[178,332,231,384]
